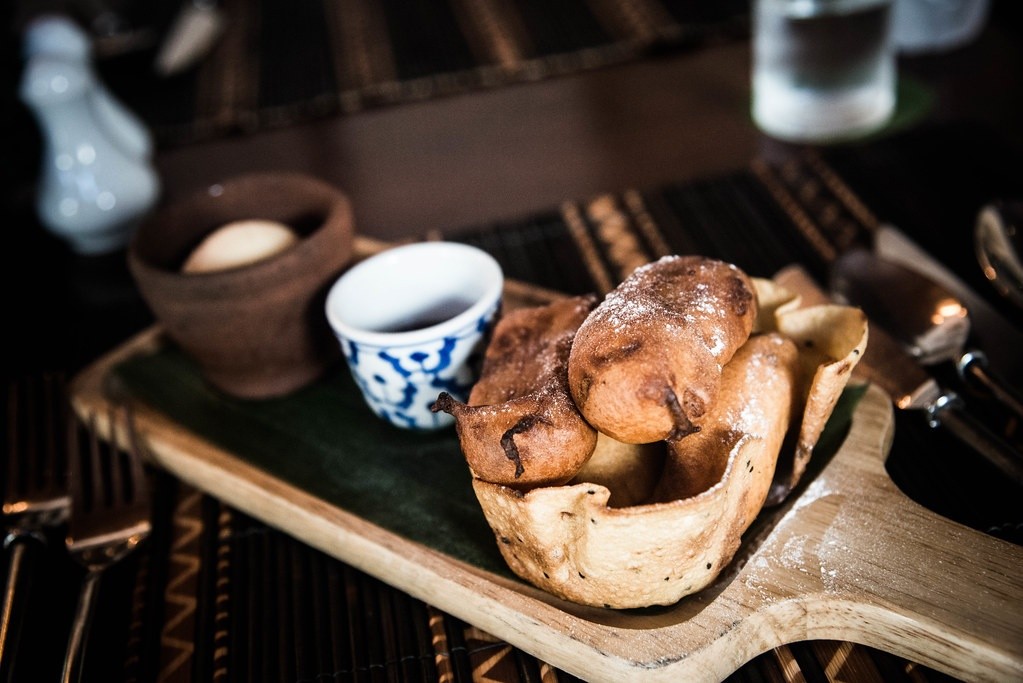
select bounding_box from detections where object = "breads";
[422,248,800,511]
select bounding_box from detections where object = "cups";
[750,2,898,142]
[323,241,504,435]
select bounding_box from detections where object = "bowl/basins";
[124,171,353,400]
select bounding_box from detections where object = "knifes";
[859,330,1023,486]
[881,229,1023,368]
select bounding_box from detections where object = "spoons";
[973,200,1023,305]
[831,250,1023,430]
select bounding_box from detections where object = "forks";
[58,406,152,683]
[0,377,75,682]
